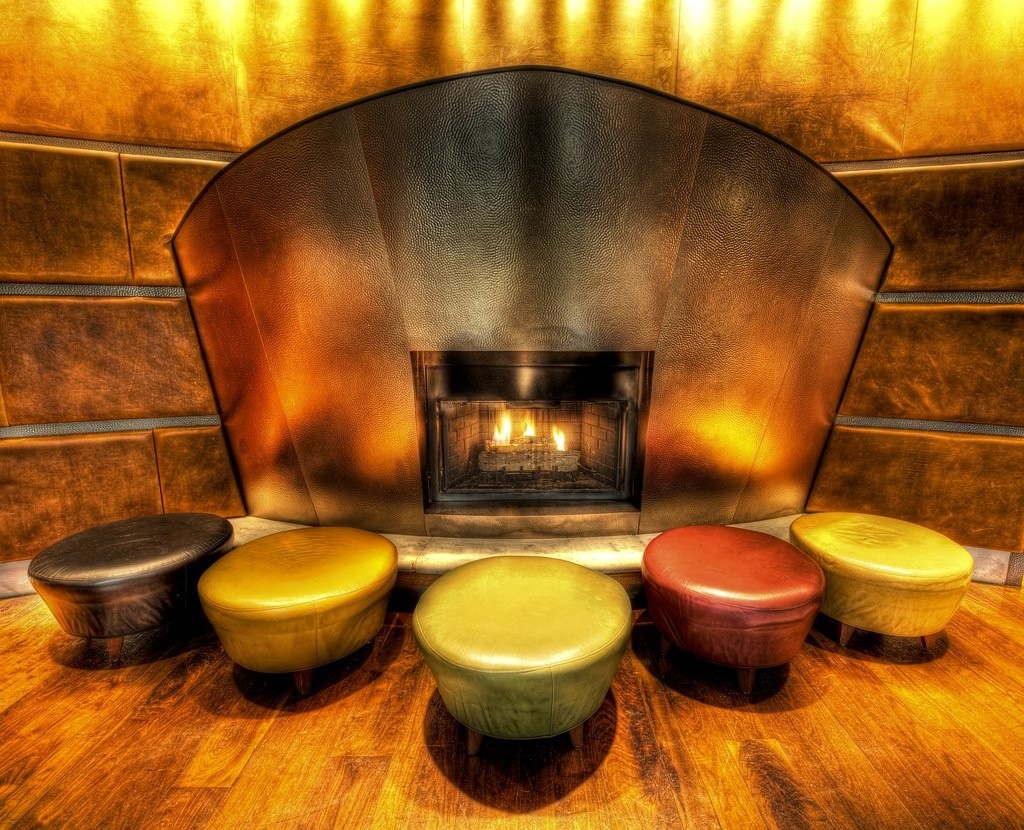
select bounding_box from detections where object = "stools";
[788,510,973,655]
[411,553,632,755]
[29,511,236,659]
[201,524,401,696]
[641,524,827,698]
